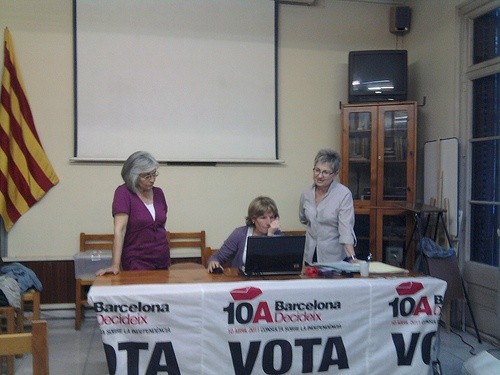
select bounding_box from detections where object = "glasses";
[312,168,336,177]
[139,173,159,180]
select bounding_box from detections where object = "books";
[349,111,407,160]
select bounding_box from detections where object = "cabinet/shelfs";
[335,100,418,270]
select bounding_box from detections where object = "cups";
[359,262,370,278]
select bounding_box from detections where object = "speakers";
[390,6,410,32]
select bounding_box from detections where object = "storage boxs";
[73,249,114,279]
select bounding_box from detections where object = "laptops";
[238,235,306,277]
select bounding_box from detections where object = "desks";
[85,264,449,375]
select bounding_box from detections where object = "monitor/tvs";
[347,49,408,104]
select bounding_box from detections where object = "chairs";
[167,230,209,270]
[20,286,42,321]
[203,246,216,271]
[0,319,50,375]
[72,232,117,331]
[0,305,17,375]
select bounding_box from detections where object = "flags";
[0,26,60,232]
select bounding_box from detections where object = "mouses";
[212,268,223,274]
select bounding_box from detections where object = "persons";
[207,194,282,273]
[298,148,357,266]
[95,149,170,276]
